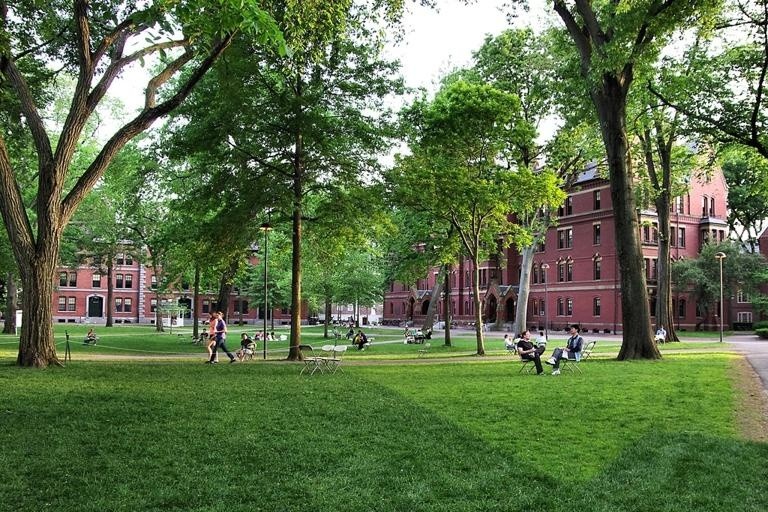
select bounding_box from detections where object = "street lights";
[257,222,273,361]
[541,263,551,339]
[714,251,726,344]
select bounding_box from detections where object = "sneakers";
[545,359,555,365]
[540,372,545,377]
[529,352,535,358]
[552,371,560,375]
[205,359,248,364]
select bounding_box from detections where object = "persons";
[545,324,584,375]
[195,328,208,343]
[504,330,547,352]
[236,333,256,362]
[255,328,277,340]
[207,310,218,361]
[346,325,354,339]
[87,328,96,345]
[209,312,236,363]
[517,330,545,375]
[403,323,432,343]
[354,330,368,351]
[656,324,667,339]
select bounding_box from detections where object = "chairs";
[352,335,373,352]
[298,344,348,376]
[562,342,596,374]
[505,336,546,374]
[417,343,430,359]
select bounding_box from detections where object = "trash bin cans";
[329,317,334,324]
[363,318,367,325]
[348,317,351,322]
[309,317,318,325]
